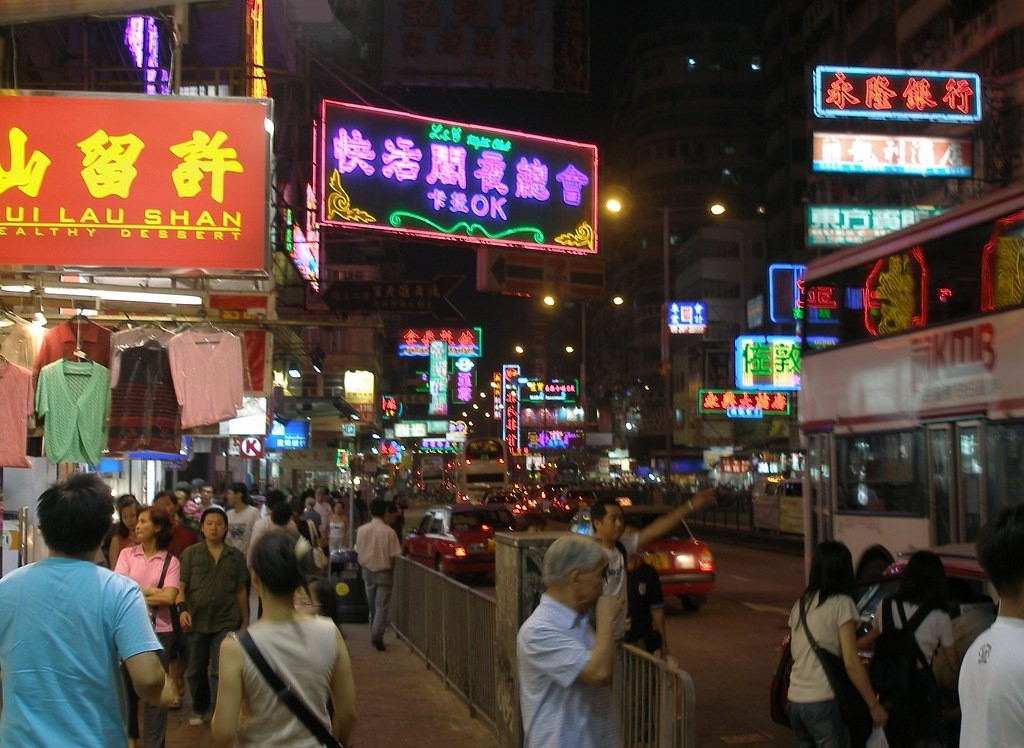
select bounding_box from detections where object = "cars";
[853,541,1001,663]
[400,501,518,581]
[565,496,715,615]
[474,479,601,532]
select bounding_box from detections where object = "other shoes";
[372,638,385,651]
[190,713,205,725]
[172,695,181,708]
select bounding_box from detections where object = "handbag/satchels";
[865,724,891,748]
[924,685,960,729]
[813,650,860,721]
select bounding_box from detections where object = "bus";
[798,189,1022,599]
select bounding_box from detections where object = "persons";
[211,530,358,748]
[583,487,717,745]
[783,540,888,748]
[113,504,180,748]
[355,498,402,650]
[179,508,248,725]
[0,469,181,748]
[959,503,1024,748]
[516,534,631,748]
[871,550,958,748]
[102,478,409,651]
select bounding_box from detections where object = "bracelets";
[176,602,188,616]
[687,500,696,513]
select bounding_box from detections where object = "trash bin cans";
[325,548,370,621]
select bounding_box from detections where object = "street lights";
[542,293,627,409]
[608,196,727,499]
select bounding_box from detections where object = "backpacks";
[868,595,932,738]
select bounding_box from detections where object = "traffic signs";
[476,244,605,302]
